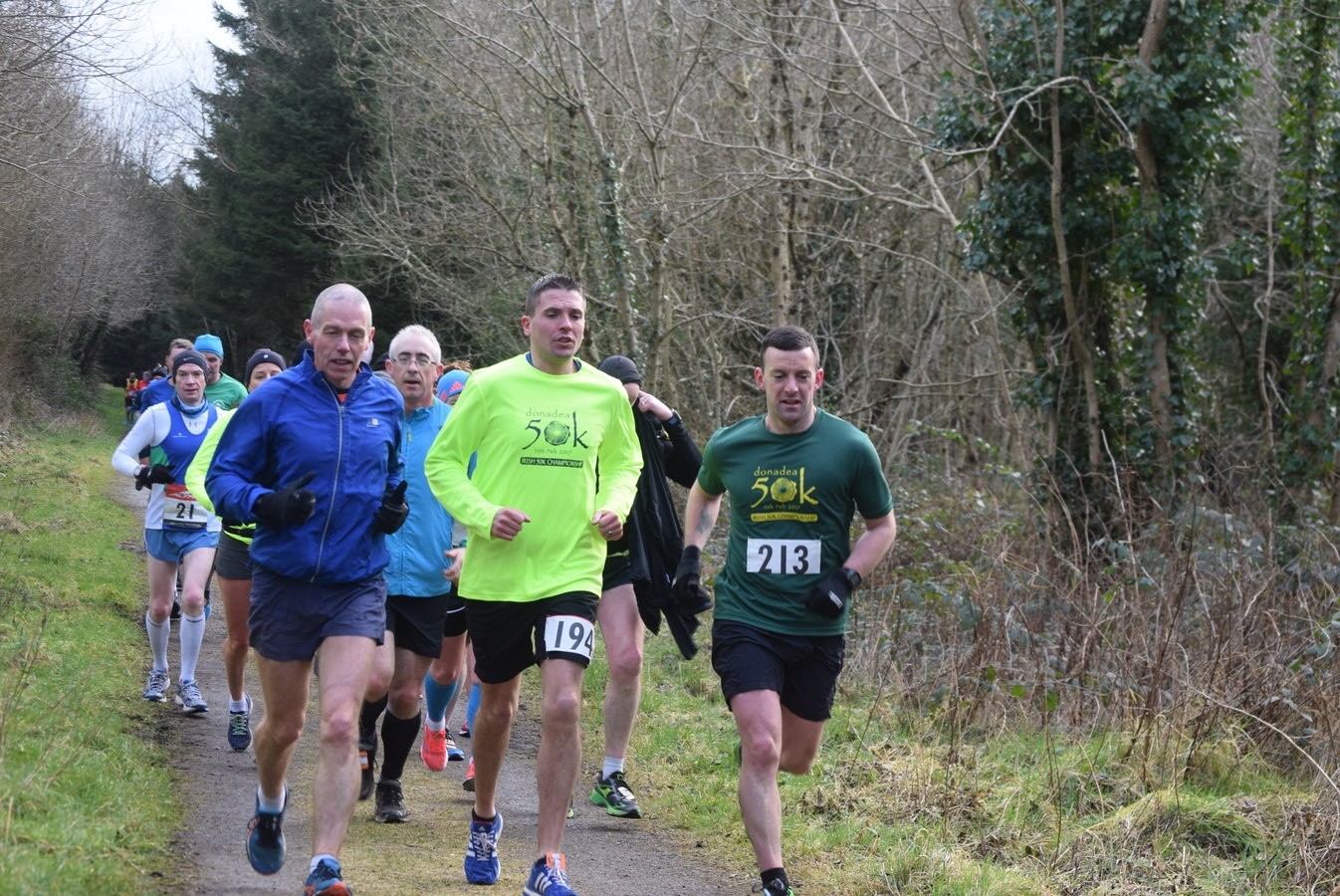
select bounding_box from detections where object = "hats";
[437,370,470,402]
[245,348,286,384]
[193,334,223,359]
[600,355,642,387]
[172,349,206,382]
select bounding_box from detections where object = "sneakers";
[522,853,578,896]
[445,726,465,760]
[460,722,469,737]
[227,693,253,750]
[246,783,288,876]
[357,733,378,800]
[761,879,794,896]
[463,758,474,790]
[303,858,352,896]
[588,770,641,818]
[141,669,171,701]
[464,810,503,885]
[175,680,209,713]
[421,719,448,770]
[375,780,409,822]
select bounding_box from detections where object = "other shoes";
[204,601,211,619]
[171,600,180,614]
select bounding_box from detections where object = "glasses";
[392,356,436,369]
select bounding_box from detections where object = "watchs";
[841,566,863,588]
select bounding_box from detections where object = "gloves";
[671,546,711,613]
[373,479,410,533]
[261,471,317,525]
[801,570,854,618]
[138,463,176,486]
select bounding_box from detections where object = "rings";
[608,530,614,536]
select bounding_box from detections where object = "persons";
[671,326,896,896]
[421,274,644,896]
[205,284,410,896]
[111,325,702,827]
